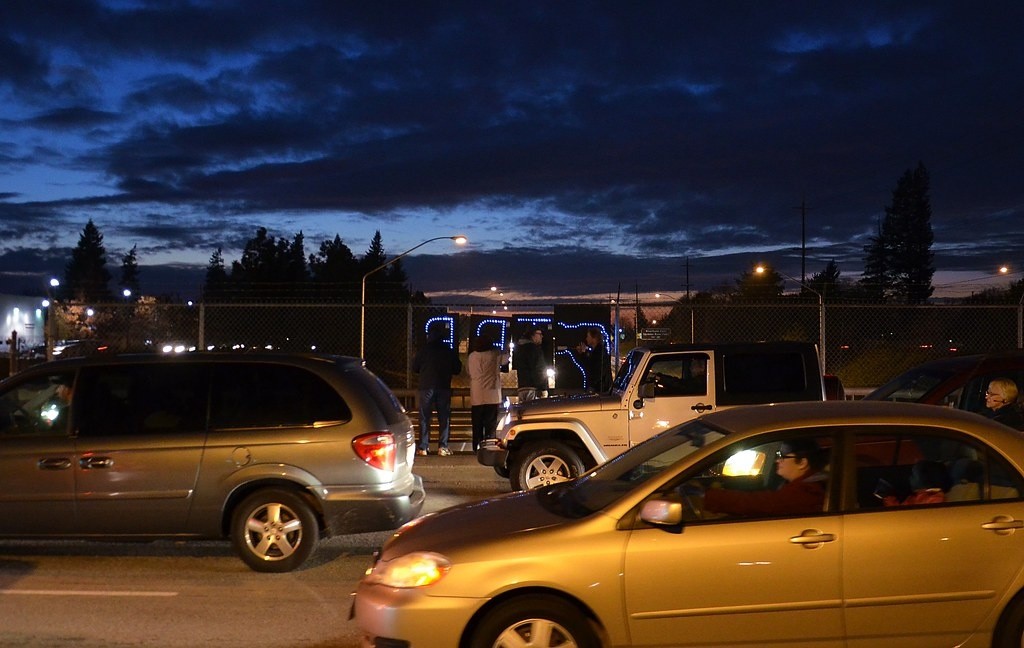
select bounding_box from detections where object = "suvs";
[475,336,846,495]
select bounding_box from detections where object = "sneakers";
[415,447,429,455]
[438,447,453,456]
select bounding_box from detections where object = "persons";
[468,336,508,453]
[412,326,463,457]
[985,378,1024,432]
[583,326,614,394]
[684,436,825,518]
[647,355,707,395]
[513,327,551,396]
[878,460,945,506]
[48,373,74,436]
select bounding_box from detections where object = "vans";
[1,349,427,574]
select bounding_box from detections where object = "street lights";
[755,265,823,369]
[361,236,468,360]
[655,293,694,345]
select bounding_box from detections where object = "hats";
[425,328,441,338]
[51,375,75,388]
[523,326,545,337]
[474,333,492,346]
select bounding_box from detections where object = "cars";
[350,401,1024,648]
[820,352,1024,465]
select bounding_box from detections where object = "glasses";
[690,362,705,368]
[536,333,543,336]
[776,451,804,459]
[986,391,1000,396]
